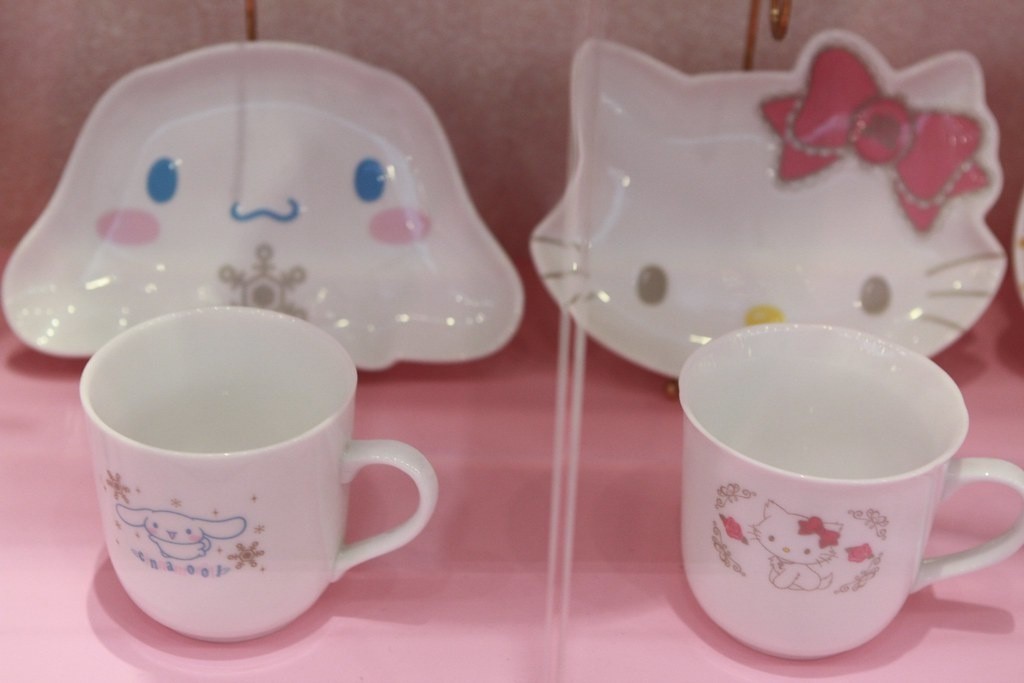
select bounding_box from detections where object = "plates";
[529,29,1007,377]
[0,38,522,374]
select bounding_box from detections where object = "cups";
[80,306,437,642]
[678,325,1024,660]
